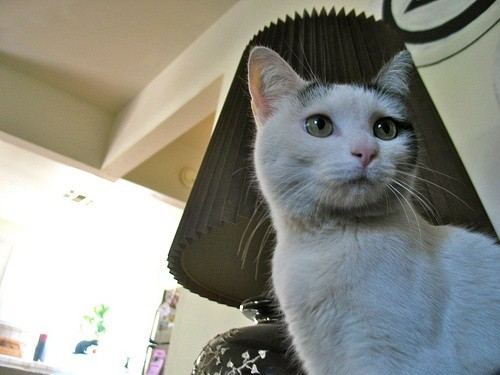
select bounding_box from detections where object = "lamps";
[167,7,500,375]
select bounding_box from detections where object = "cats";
[231,35,500,375]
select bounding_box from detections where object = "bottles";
[33,334,48,362]
